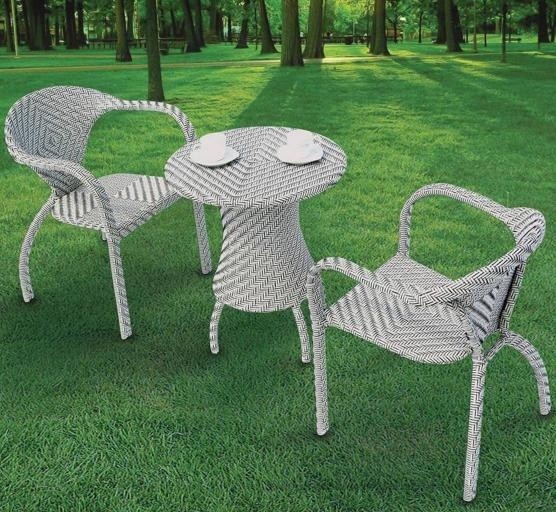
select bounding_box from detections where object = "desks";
[164,126,348,365]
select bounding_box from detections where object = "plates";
[276,143,324,165]
[190,146,240,168]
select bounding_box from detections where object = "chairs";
[302,181,551,503]
[4,85,213,342]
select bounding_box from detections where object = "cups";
[192,133,227,162]
[286,129,323,159]
